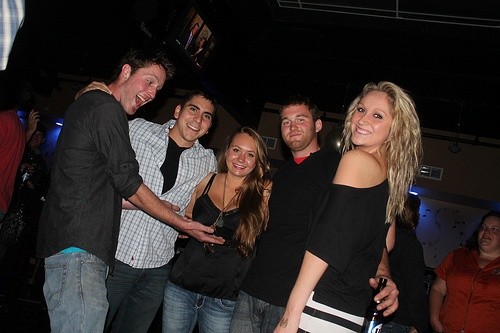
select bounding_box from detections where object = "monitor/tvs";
[174,5,216,71]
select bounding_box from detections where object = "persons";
[74,80,220,333]
[35,48,226,333]
[380,192,426,333]
[181,23,209,57]
[0,70,27,227]
[428,211,500,333]
[161,126,272,333]
[0,109,47,248]
[227,98,399,333]
[272,80,424,333]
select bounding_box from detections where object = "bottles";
[362,278,387,333]
[19,158,34,189]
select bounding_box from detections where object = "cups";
[425,279,433,294]
